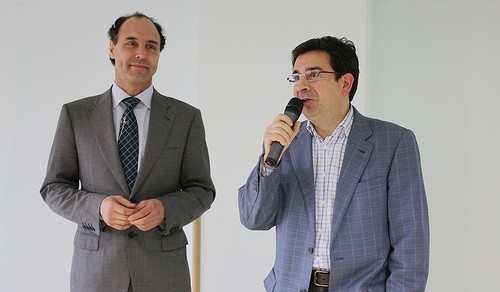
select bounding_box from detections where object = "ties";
[117,98,139,195]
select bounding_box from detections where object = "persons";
[238,37,430,292]
[40,12,216,292]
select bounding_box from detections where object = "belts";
[310,270,330,288]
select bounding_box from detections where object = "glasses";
[286,70,346,85]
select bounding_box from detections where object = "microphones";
[266,98,303,166]
